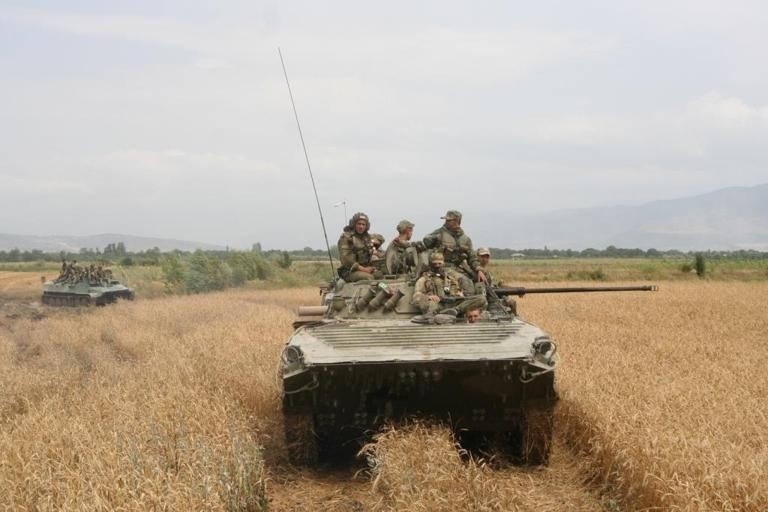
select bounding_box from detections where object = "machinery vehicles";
[275,45,659,469]
[41,267,135,308]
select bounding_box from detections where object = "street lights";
[333,201,347,226]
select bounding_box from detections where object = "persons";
[474,247,517,316]
[466,306,483,324]
[384,218,419,276]
[410,252,488,325]
[335,212,388,282]
[53,258,120,288]
[392,210,488,285]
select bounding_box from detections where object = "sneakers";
[440,308,457,316]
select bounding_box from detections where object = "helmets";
[429,252,444,264]
[349,213,370,230]
[374,234,384,244]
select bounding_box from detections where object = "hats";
[440,210,462,220]
[397,220,414,232]
[477,248,490,256]
